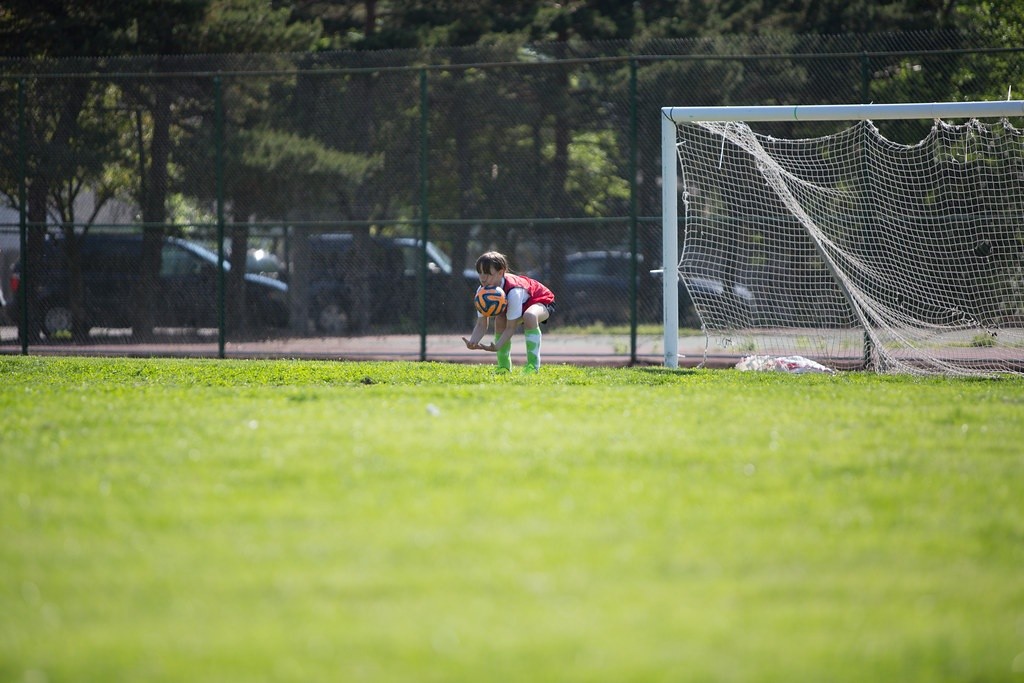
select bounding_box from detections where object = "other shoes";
[492,367,512,375]
[524,365,538,375]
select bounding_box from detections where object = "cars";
[517,249,761,332]
[6,226,291,341]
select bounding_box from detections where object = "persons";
[462,250,556,375]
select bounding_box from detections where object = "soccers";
[474,285,507,317]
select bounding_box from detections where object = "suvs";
[293,229,485,337]
